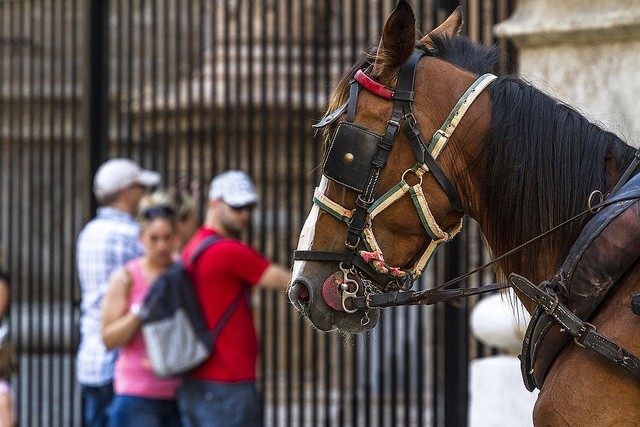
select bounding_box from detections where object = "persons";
[1,276,16,427]
[75,158,162,426]
[180,171,292,427]
[100,206,184,427]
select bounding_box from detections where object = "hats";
[93,158,162,202]
[208,169,258,210]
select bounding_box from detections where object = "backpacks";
[139,236,248,377]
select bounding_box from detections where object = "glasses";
[142,205,176,220]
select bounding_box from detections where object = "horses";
[287,0,639,426]
